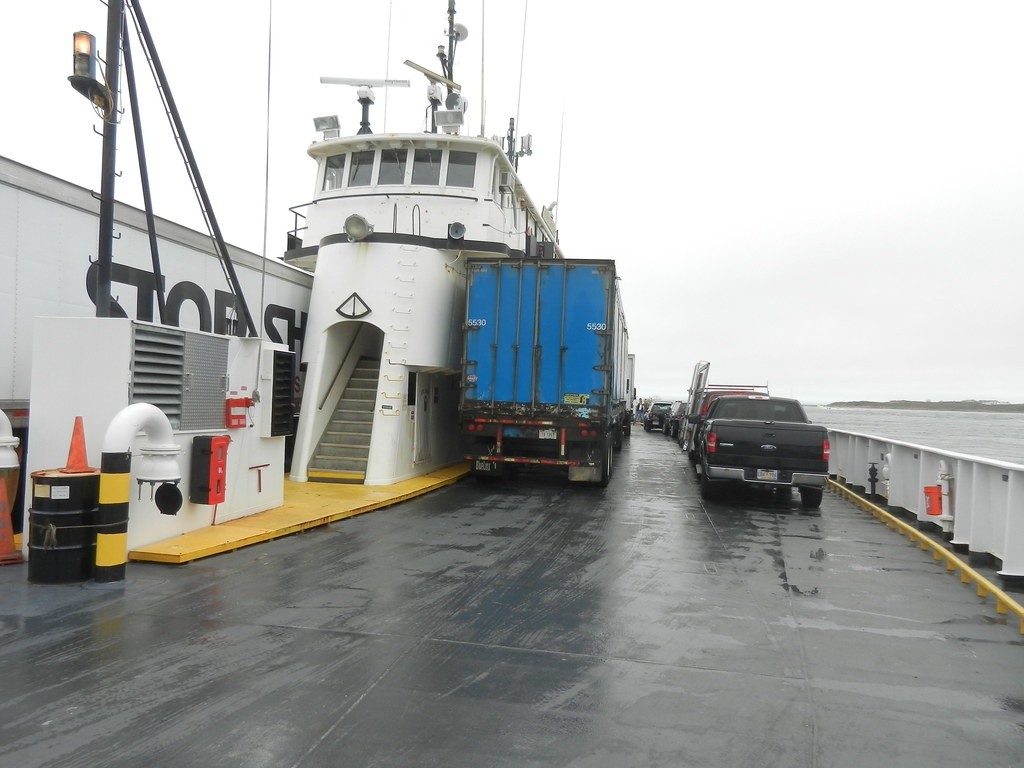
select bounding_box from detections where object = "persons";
[632,397,650,425]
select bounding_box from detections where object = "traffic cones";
[0,477,23,565]
[57,416,96,472]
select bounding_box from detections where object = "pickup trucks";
[688,394,831,508]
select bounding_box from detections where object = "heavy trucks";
[460,256,627,488]
[624,354,636,438]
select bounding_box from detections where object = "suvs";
[662,400,688,437]
[644,401,672,432]
